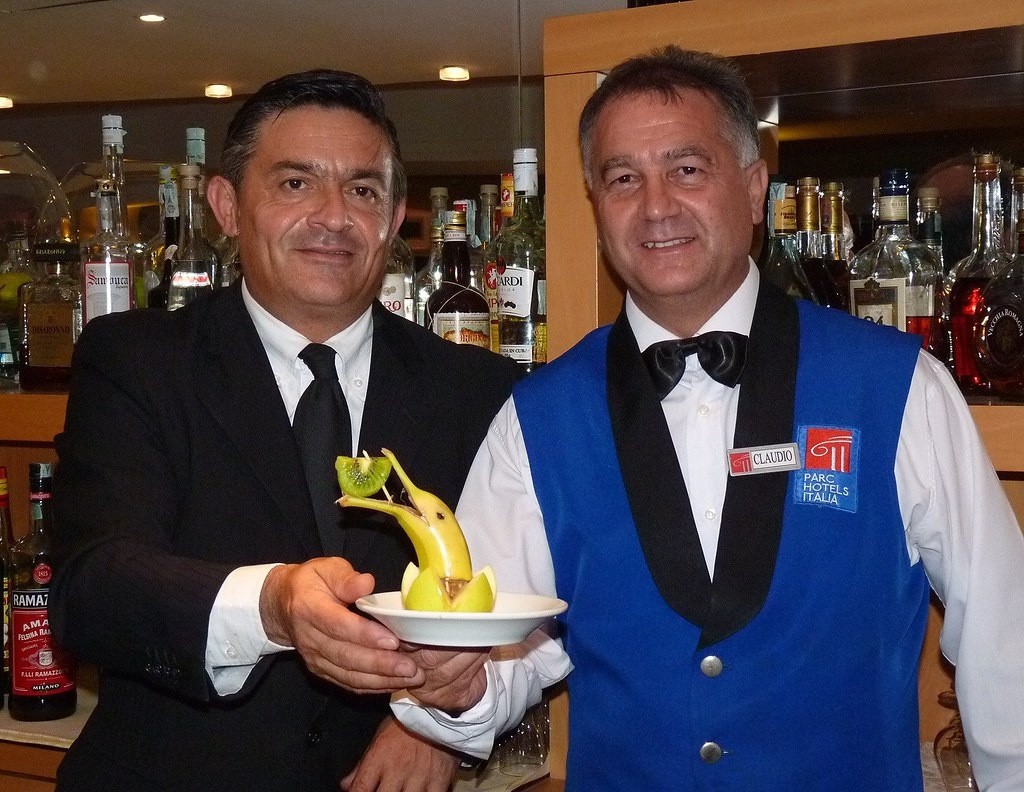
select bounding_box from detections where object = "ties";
[292,343,352,558]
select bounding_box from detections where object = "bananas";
[335,448,472,597]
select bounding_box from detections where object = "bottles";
[0,466,18,710]
[760,152,1024,406]
[0,104,548,381]
[9,461,80,722]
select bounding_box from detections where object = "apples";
[401,562,497,613]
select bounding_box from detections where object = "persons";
[47,69,522,792]
[390,46,1024,792]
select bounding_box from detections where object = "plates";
[352,592,570,647]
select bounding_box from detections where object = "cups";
[495,695,552,778]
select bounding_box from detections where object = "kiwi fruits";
[335,455,394,498]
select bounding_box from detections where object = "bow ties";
[640,331,749,401]
